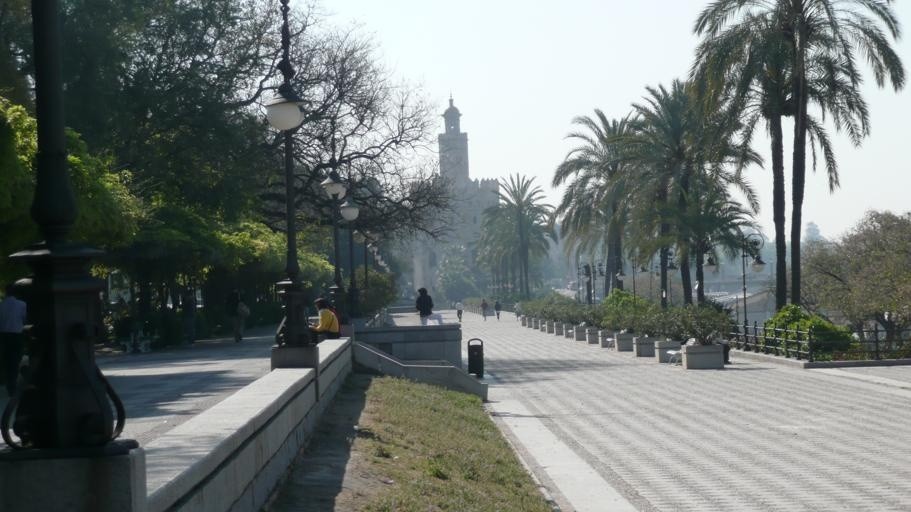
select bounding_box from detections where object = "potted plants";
[516,285,734,369]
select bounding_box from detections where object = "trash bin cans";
[468,338,483,379]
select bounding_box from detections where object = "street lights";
[263,0,317,349]
[321,120,391,299]
[742,233,767,351]
[576,249,717,305]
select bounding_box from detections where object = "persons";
[322,299,338,316]
[515,300,522,321]
[495,301,502,319]
[0,284,28,398]
[226,286,248,342]
[456,300,463,322]
[182,292,199,342]
[481,298,489,321]
[309,298,341,341]
[416,287,444,327]
